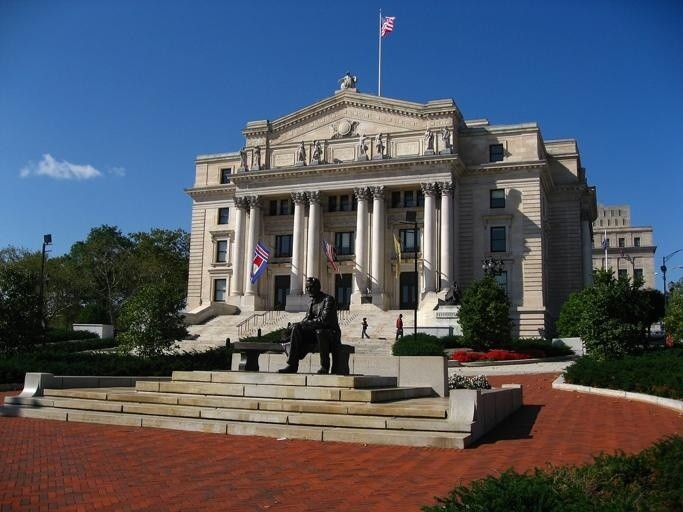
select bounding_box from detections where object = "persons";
[395,314,402,338]
[451,282,462,305]
[276,277,341,373]
[360,318,369,339]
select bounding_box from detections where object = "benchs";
[231,339,356,377]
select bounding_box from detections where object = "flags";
[322,239,342,278]
[393,237,400,278]
[601,235,608,253]
[381,14,396,38]
[250,240,270,284]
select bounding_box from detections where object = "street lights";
[481,255,505,287]
[39,233,52,332]
[660,249,683,319]
